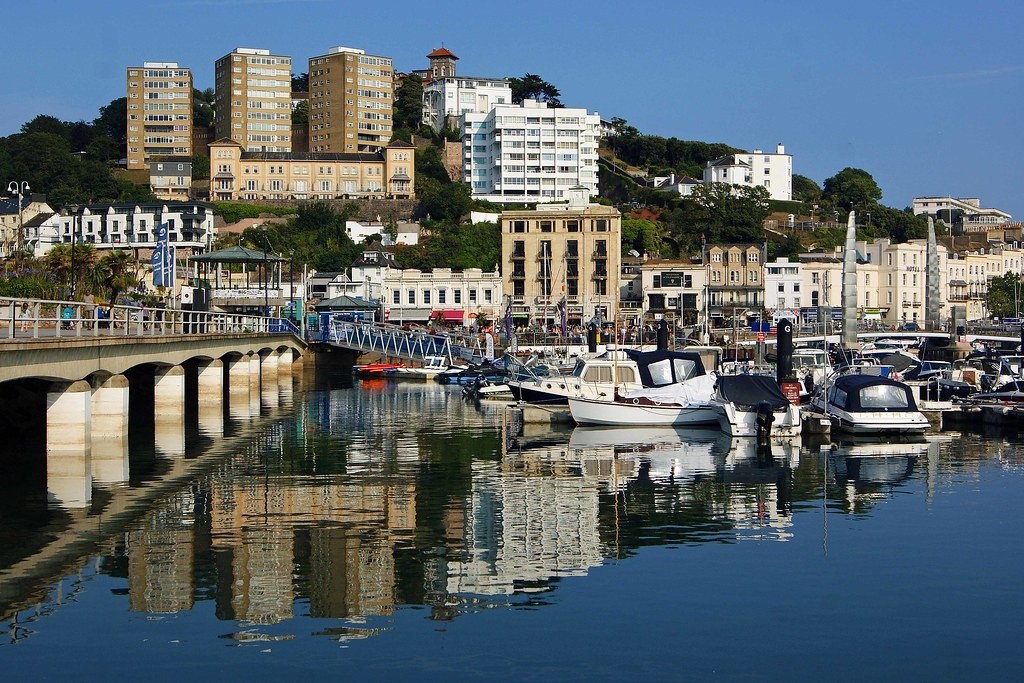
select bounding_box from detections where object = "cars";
[898,323,920,330]
[877,324,891,331]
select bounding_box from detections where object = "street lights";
[289,248,295,319]
[7,180,30,252]
[261,221,270,306]
[64,205,86,299]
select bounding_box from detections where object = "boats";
[506,342,710,411]
[564,424,727,486]
[827,435,931,490]
[721,337,1024,419]
[811,376,931,435]
[707,375,804,438]
[565,373,721,426]
[352,355,559,407]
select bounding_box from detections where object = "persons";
[18,302,33,333]
[142,302,150,330]
[83,290,95,331]
[154,296,170,330]
[62,295,75,308]
[485,295,1024,368]
[98,305,106,319]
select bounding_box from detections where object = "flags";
[168,248,174,288]
[151,246,163,286]
[157,223,168,286]
